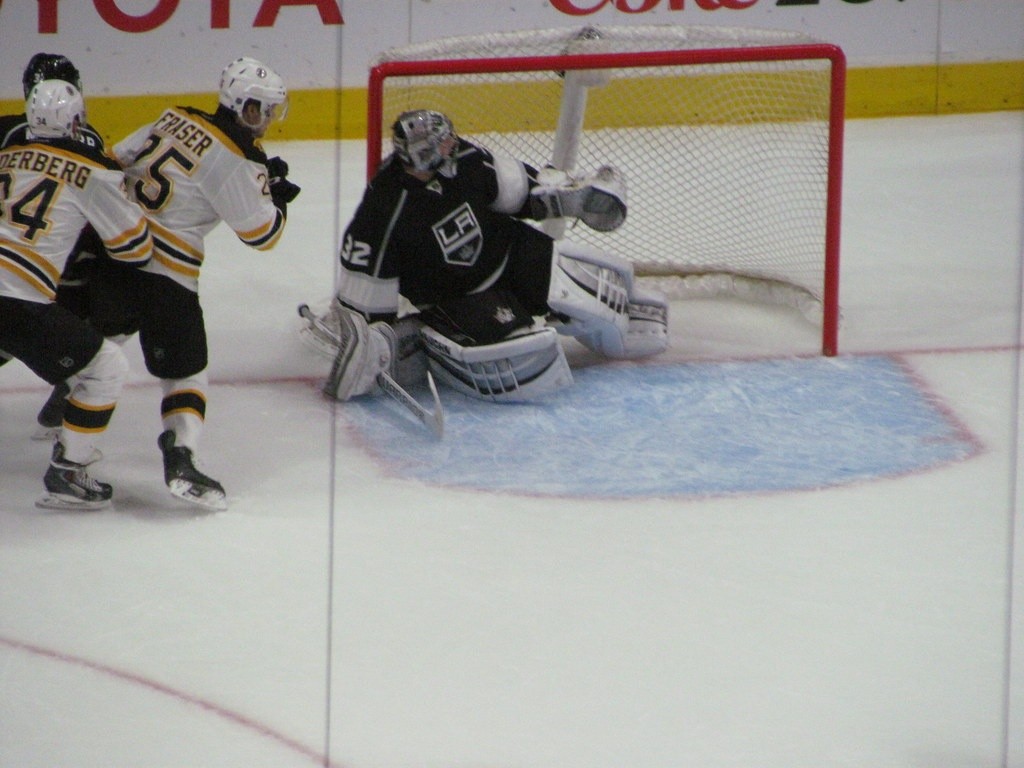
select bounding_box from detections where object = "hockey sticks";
[296,302,446,442]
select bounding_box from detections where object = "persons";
[313,109,668,402]
[1,50,302,516]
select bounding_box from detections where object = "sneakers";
[29,382,71,441]
[33,434,114,509]
[157,429,228,512]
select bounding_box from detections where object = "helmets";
[22,52,80,102]
[218,57,287,130]
[25,79,85,139]
[390,109,460,176]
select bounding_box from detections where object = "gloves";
[265,156,301,205]
